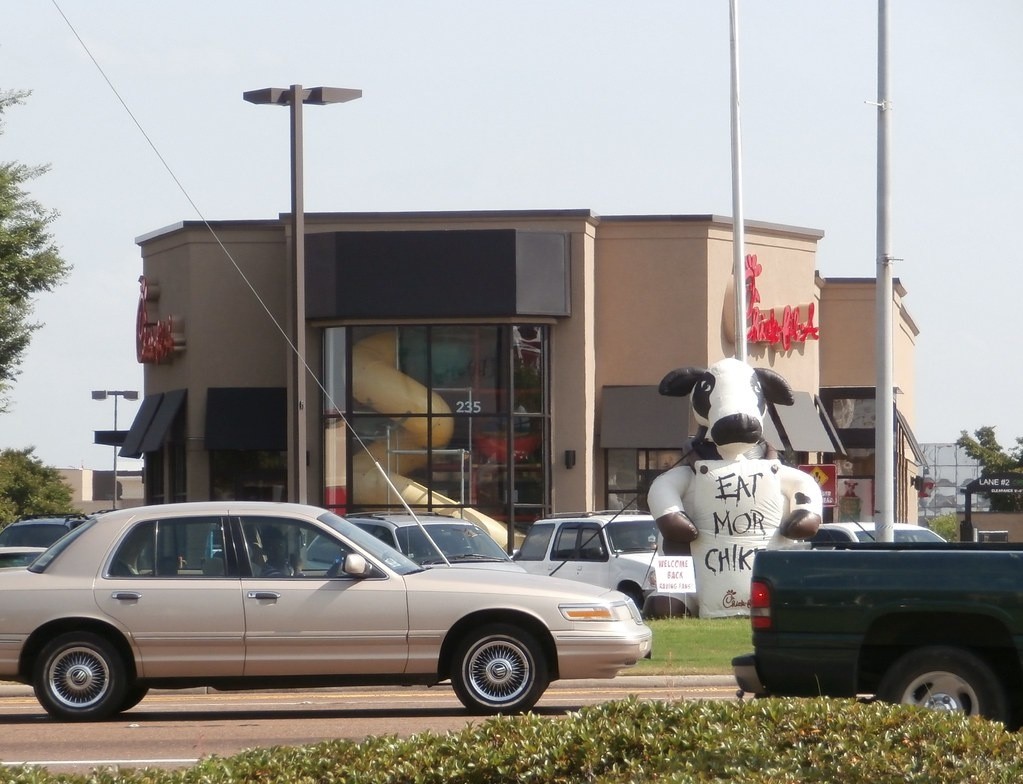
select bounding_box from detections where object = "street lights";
[241,84,364,504]
[92,390,139,511]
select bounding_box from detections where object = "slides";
[351,332,528,554]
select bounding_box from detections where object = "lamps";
[911,476,923,490]
[565,450,575,469]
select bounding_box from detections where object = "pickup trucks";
[731,541,1023,736]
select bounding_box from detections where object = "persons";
[119,545,141,575]
[259,527,304,578]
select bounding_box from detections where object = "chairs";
[586,546,604,559]
[110,560,138,575]
[203,544,265,578]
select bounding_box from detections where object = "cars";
[0,501,652,722]
[811,521,947,541]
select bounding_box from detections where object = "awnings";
[815,394,848,456]
[599,384,788,451]
[140,388,187,453]
[118,393,163,459]
[897,410,930,468]
[774,390,837,453]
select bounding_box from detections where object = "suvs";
[511,510,659,613]
[301,511,527,575]
[0,514,85,568]
